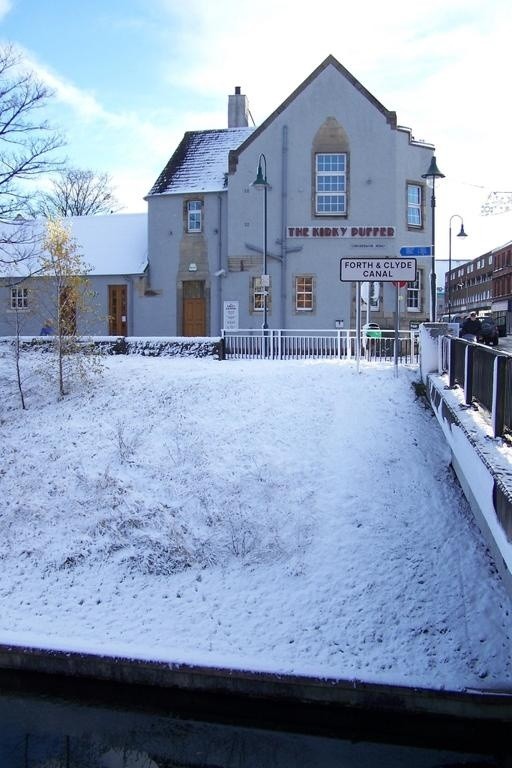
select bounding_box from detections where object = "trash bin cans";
[363,323,382,357]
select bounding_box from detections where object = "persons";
[461,312,480,343]
[40,318,58,337]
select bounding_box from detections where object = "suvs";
[440,314,507,348]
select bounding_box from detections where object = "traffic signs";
[400,246,432,257]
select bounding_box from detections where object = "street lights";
[250,153,268,330]
[448,215,467,325]
[421,155,445,319]
